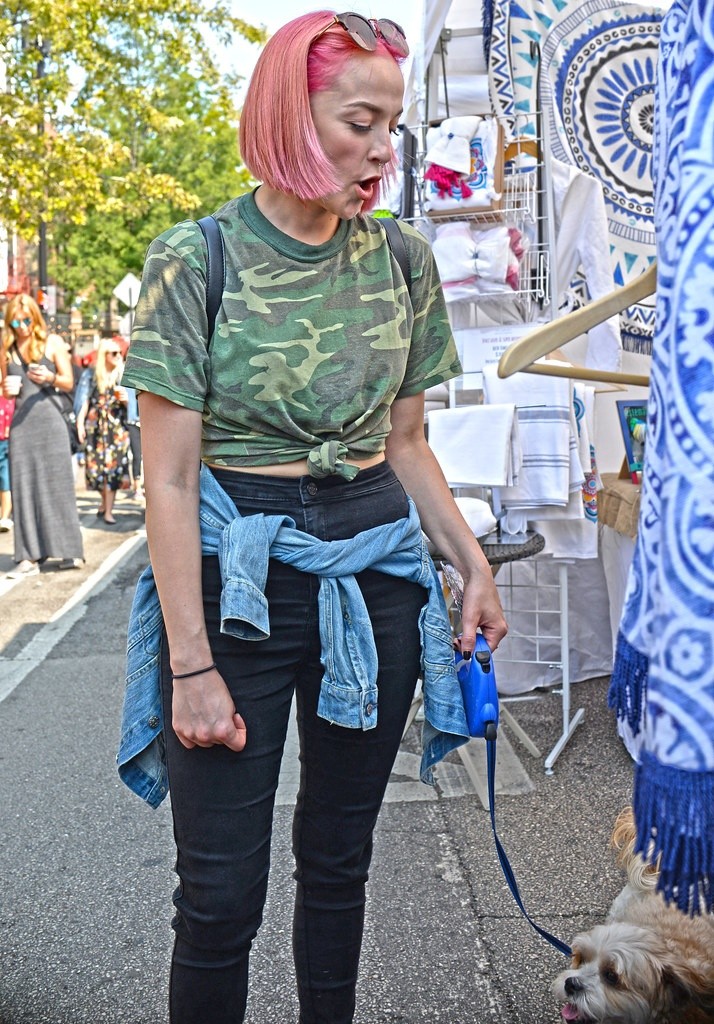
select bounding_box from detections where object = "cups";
[4,374,21,396]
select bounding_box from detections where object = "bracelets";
[170,662,217,679]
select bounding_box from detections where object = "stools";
[400,524,548,818]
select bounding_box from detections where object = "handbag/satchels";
[62,410,81,456]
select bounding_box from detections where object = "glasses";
[9,317,32,329]
[311,11,409,58]
[110,350,122,356]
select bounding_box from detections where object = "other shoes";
[96,502,117,525]
[5,560,40,578]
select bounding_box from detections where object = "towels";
[386,107,604,563]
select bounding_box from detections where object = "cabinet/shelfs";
[381,105,588,777]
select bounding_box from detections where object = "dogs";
[550,806,714,1024]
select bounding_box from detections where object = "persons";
[118,10,504,1023]
[76,336,130,524]
[72,362,144,504]
[1,292,86,579]
[60,343,80,456]
[0,319,15,531]
